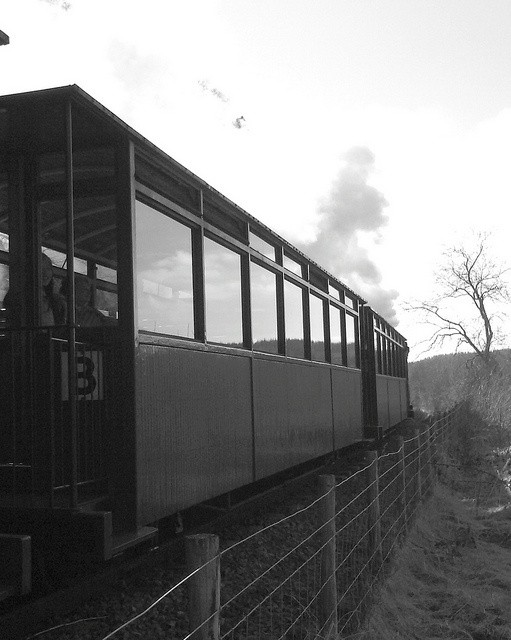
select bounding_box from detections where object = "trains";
[0,83,414,600]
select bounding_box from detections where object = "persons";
[40,252,68,334]
[75,276,107,327]
